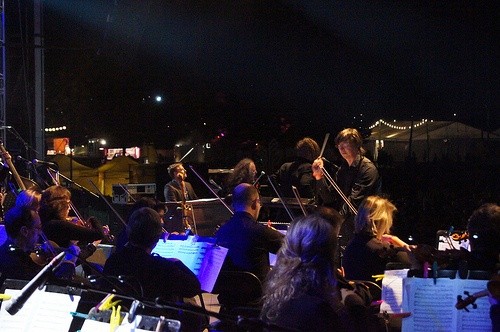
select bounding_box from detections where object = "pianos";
[163,196,234,237]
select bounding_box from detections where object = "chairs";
[0,175,477,332]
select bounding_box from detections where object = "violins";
[66,217,115,242]
[454,272,500,312]
[382,233,417,250]
[29,238,61,266]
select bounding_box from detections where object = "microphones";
[0,125,12,130]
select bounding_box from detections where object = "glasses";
[337,142,351,150]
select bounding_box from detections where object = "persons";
[314,206,345,277]
[312,128,380,228]
[437,204,500,270]
[164,163,197,231]
[213,183,284,317]
[118,197,168,251]
[274,138,319,198]
[221,158,256,197]
[0,151,110,288]
[259,215,372,332]
[344,197,417,281]
[101,207,202,303]
